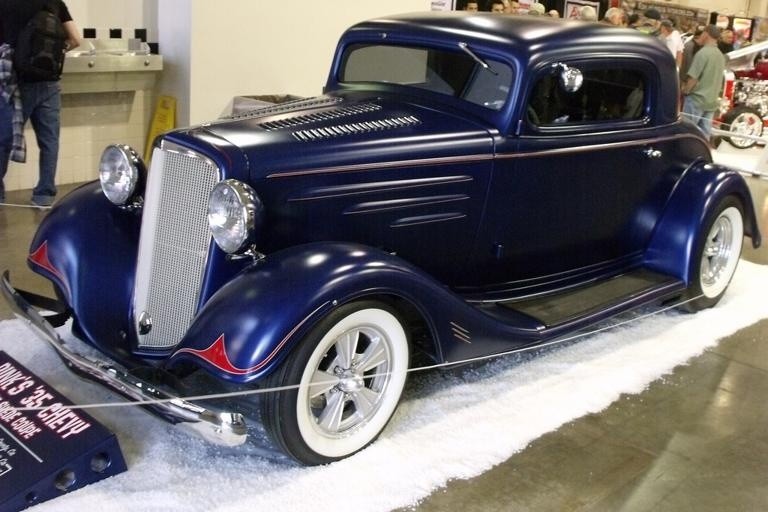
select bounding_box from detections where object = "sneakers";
[29,193,53,212]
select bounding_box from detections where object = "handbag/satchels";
[12,9,69,83]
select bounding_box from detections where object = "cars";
[2,11,759,466]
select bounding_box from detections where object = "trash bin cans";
[230,92,303,112]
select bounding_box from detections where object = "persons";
[462,0,745,145]
[0,0,82,210]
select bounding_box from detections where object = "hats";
[644,9,660,19]
[528,4,544,14]
